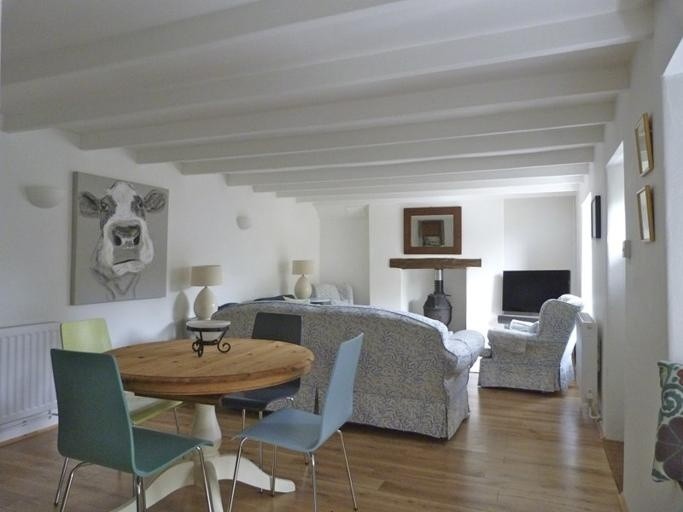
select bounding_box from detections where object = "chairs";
[50,348,213,512]
[53,317,184,508]
[227,333,364,511]
[222,311,311,465]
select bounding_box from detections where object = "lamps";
[293,260,313,300]
[191,265,223,321]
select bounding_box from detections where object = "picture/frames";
[403,206,463,254]
[590,195,601,238]
[633,111,654,177]
[636,185,654,242]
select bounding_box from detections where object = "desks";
[389,258,481,324]
[103,337,315,511]
[497,313,540,326]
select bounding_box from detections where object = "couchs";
[312,283,353,306]
[478,294,584,392]
[211,301,486,440]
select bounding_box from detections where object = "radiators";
[576,311,599,405]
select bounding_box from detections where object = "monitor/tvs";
[502,270,571,316]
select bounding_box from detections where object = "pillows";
[651,360,683,482]
